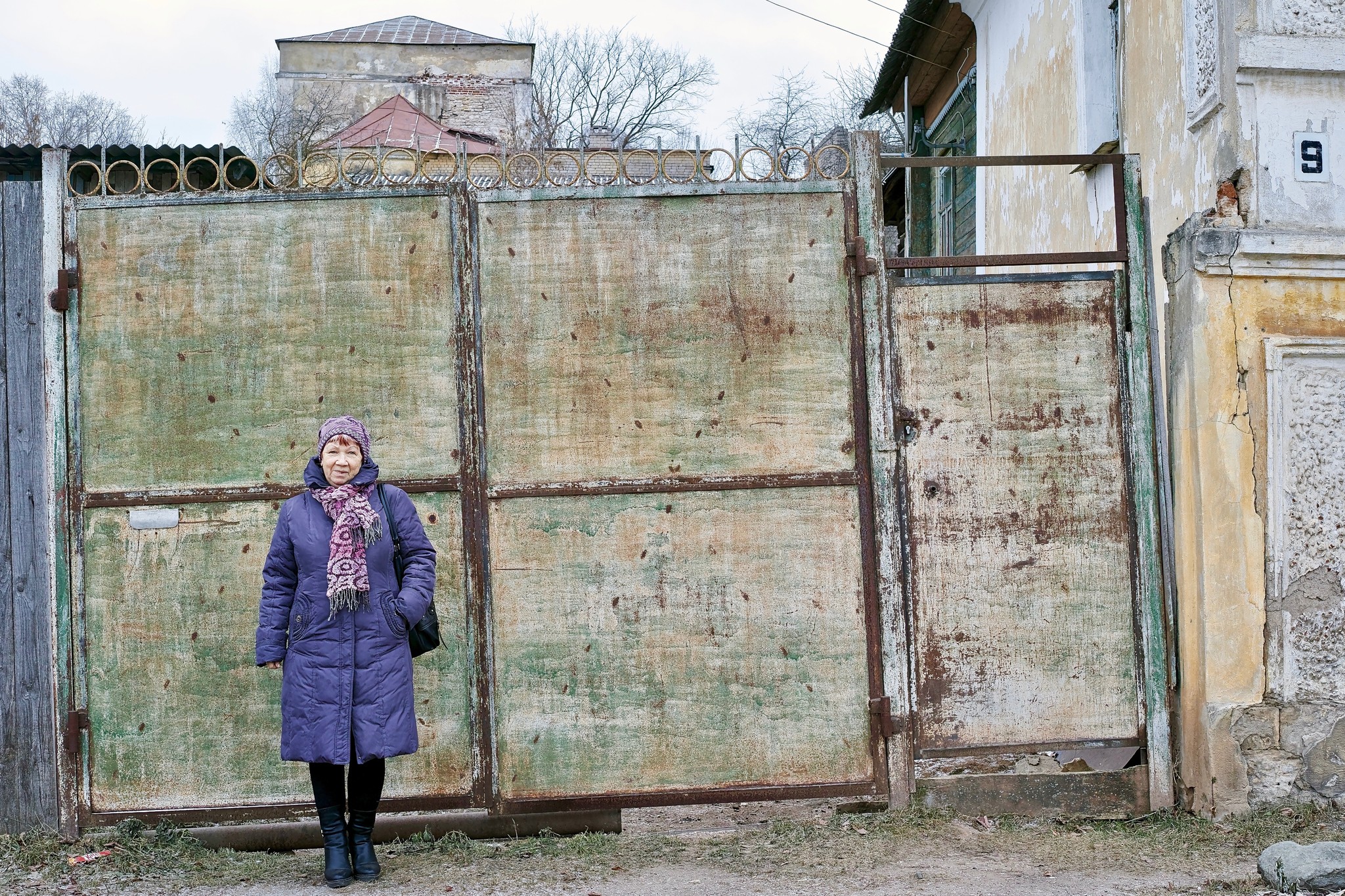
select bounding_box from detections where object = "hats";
[316,417,370,463]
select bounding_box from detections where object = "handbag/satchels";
[411,601,440,656]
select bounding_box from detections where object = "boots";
[316,804,353,887]
[348,807,382,883]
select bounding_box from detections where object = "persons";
[255,415,440,891]
